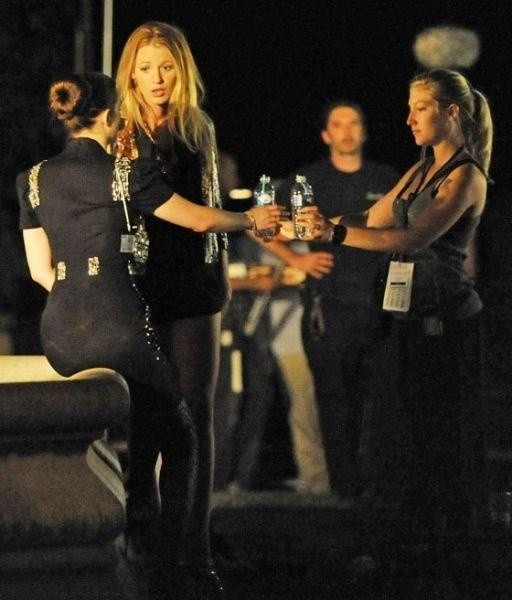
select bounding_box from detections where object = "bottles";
[254,175,276,240]
[291,176,314,237]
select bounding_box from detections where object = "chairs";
[0,355,128,576]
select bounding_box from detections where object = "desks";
[221,276,297,492]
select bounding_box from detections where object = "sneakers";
[280,477,335,498]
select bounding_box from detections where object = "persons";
[8,14,494,600]
